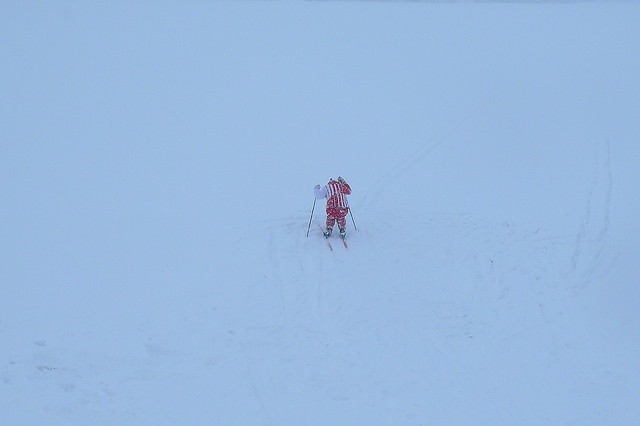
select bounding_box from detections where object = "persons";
[314,177,352,238]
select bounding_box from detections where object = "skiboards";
[317,221,347,251]
